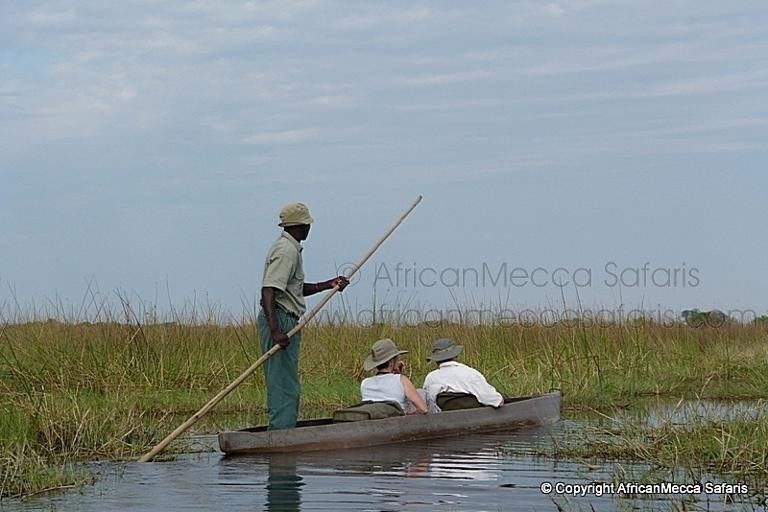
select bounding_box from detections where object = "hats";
[364,339,409,369]
[277,202,314,226]
[425,339,464,363]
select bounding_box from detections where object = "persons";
[357,336,428,418]
[253,200,353,434]
[417,337,507,412]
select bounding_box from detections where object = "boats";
[217,389,564,456]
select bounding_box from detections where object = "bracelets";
[315,283,321,292]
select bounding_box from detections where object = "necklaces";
[376,372,390,374]
